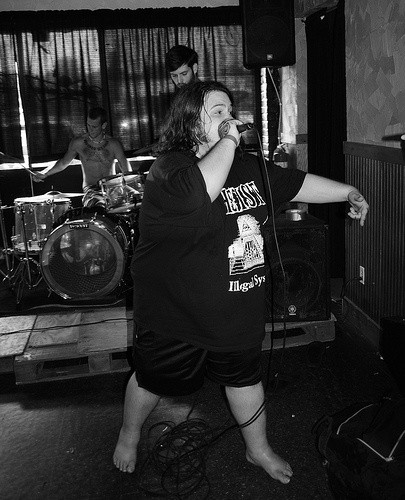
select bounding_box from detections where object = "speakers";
[261,213,331,323]
[239,0,296,69]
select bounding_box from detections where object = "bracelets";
[224,135,238,148]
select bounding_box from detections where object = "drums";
[39,206,128,300]
[100,173,142,209]
[13,196,71,255]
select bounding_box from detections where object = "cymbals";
[39,190,85,198]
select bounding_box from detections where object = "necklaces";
[84,136,110,150]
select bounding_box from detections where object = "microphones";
[237,123,254,133]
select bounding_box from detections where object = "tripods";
[1,203,42,303]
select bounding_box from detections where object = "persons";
[32,106,129,193]
[165,44,202,89]
[113,82,370,483]
